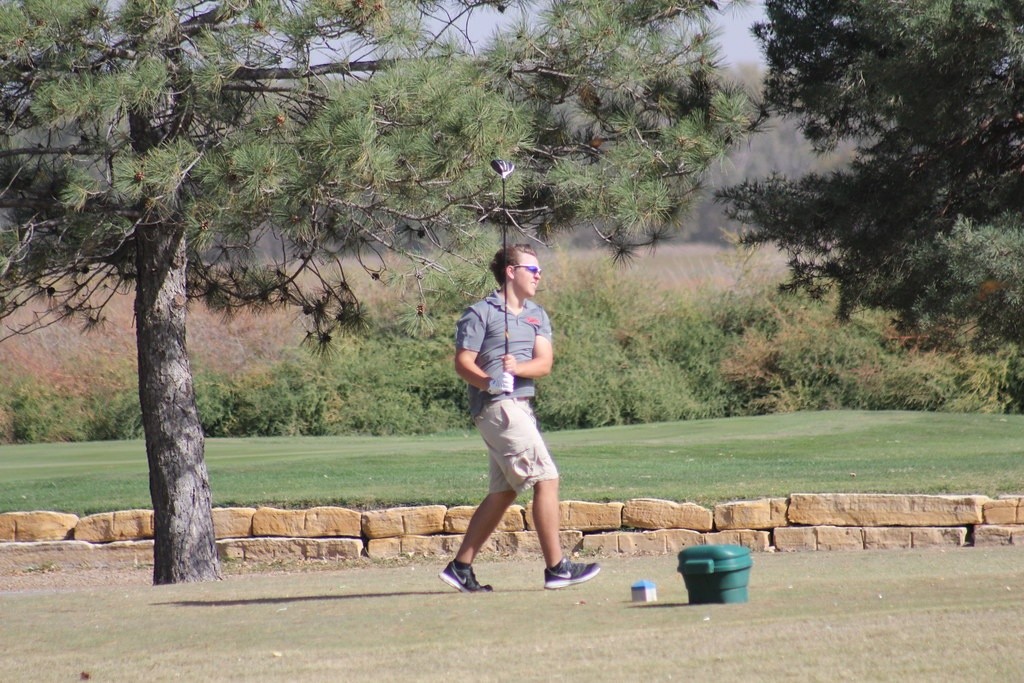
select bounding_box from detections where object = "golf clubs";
[491,158,516,396]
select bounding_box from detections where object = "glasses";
[512,265,541,275]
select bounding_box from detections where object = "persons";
[438,245,602,593]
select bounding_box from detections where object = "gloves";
[487,372,514,395]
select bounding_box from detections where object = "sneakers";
[438,560,492,592]
[544,557,601,589]
